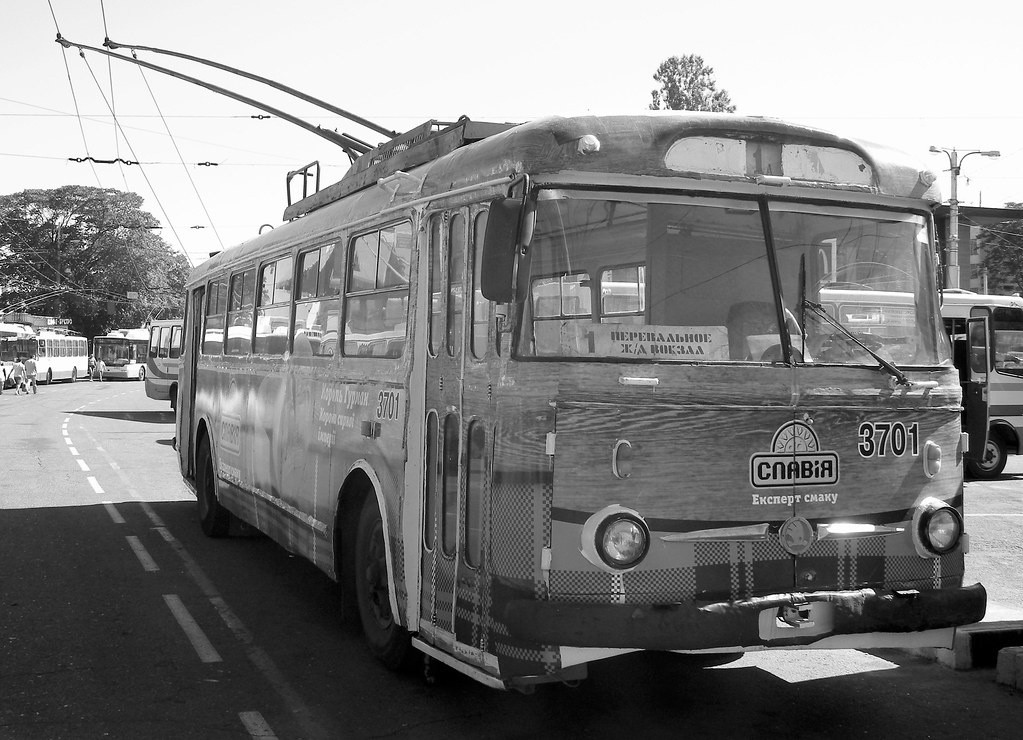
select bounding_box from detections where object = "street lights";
[931,140,1001,294]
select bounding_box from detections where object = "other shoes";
[34,392,36,394]
[26,392,29,394]
[90,380,93,381]
[15,390,19,395]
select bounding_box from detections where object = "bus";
[534,279,1023,479]
[0,320,37,387]
[142,317,184,412]
[93,327,152,382]
[52,34,989,712]
[35,332,89,385]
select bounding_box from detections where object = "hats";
[29,354,33,357]
[98,358,102,360]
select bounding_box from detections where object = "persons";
[8,358,26,395]
[109,350,114,358]
[96,358,107,382]
[24,355,38,394]
[88,354,96,382]
